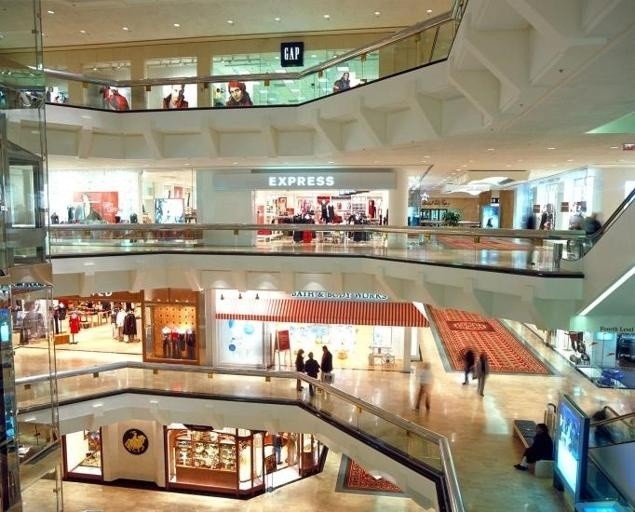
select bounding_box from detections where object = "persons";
[46,87,53,103]
[66,204,74,224]
[538,203,555,232]
[459,349,476,385]
[171,326,178,359]
[99,84,111,111]
[23,300,48,339]
[186,325,196,361]
[475,352,490,397]
[320,344,334,396]
[285,432,297,466]
[334,71,350,94]
[296,349,306,392]
[272,432,285,465]
[69,312,81,334]
[161,326,172,358]
[306,352,321,397]
[226,81,255,108]
[82,193,93,219]
[178,327,186,360]
[161,83,190,110]
[585,211,603,245]
[513,423,553,471]
[414,363,434,412]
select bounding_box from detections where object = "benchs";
[512,420,555,479]
